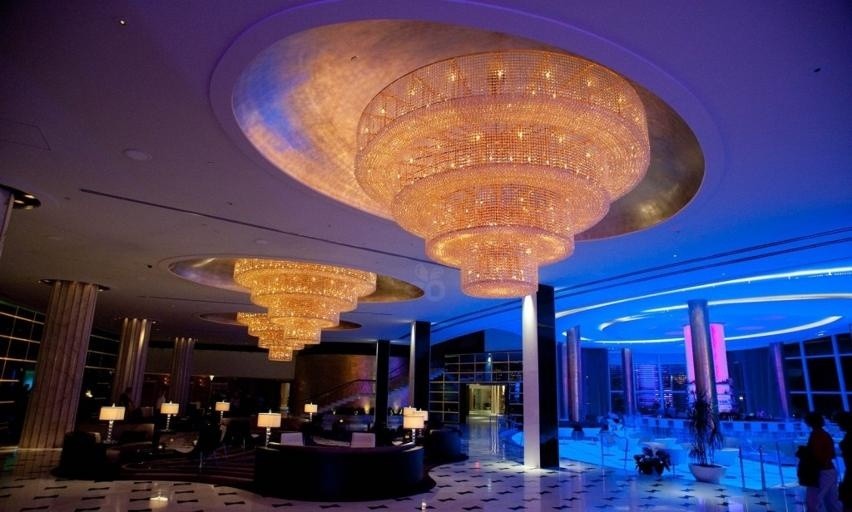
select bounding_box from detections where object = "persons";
[219,390,266,416]
[117,386,136,409]
[155,387,167,433]
[831,410,852,512]
[795,410,843,512]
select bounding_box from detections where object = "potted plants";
[634,389,727,482]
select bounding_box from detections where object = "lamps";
[257,409,282,446]
[304,403,318,422]
[161,400,180,432]
[234,256,378,363]
[215,401,230,423]
[99,403,126,444]
[354,49,650,299]
[402,406,429,443]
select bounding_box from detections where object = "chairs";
[54,431,118,480]
